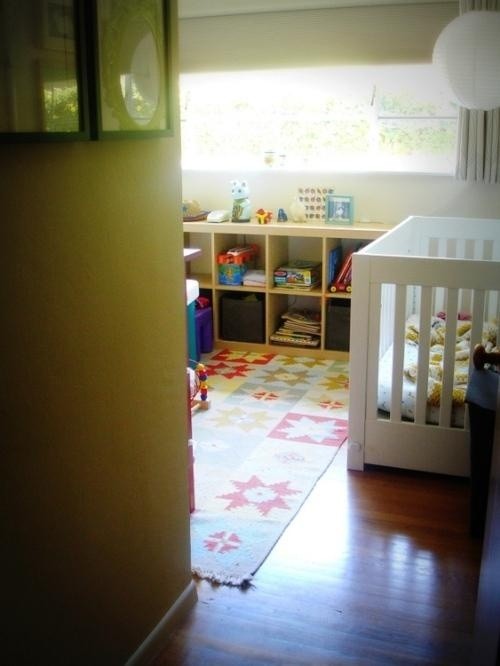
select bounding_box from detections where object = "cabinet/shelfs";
[183,222,397,362]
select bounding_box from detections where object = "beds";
[346,213,499,481]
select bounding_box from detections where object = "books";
[269,244,362,347]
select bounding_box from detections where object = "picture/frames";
[0,0,177,147]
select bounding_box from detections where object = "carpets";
[189,350,349,586]
